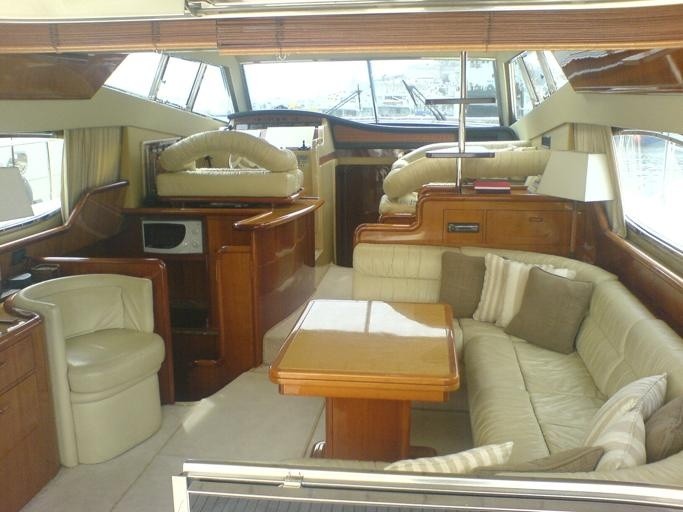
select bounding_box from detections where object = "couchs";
[284,243,683,489]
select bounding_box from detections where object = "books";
[474,180,510,191]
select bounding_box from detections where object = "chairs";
[10,274,166,468]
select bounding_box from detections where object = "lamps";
[537,150,615,259]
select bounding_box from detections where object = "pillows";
[644,395,683,464]
[439,251,596,355]
[384,442,513,474]
[579,397,647,471]
[589,373,668,425]
[472,446,604,473]
